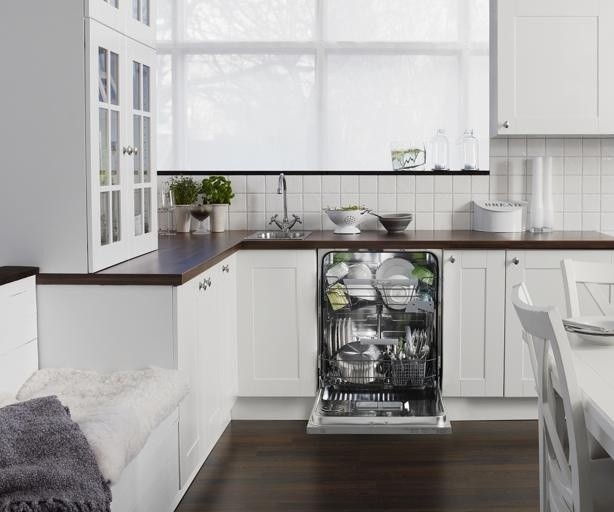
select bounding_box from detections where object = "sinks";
[244,228,313,240]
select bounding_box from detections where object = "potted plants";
[168,176,201,232]
[201,175,236,232]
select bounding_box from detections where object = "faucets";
[269,172,302,229]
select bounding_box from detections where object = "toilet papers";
[528,153,554,233]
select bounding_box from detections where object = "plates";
[563,316,614,344]
[327,316,353,358]
[326,253,435,311]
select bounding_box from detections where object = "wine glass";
[190,193,212,235]
[160,190,178,236]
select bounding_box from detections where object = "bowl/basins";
[378,213,414,234]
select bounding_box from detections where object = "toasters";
[470,199,525,233]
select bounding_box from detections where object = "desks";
[546,316,614,464]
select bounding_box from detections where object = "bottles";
[462,127,480,170]
[527,156,553,229]
[429,127,450,171]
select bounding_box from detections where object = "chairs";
[512,282,614,512]
[560,258,613,321]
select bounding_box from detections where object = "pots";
[329,339,388,385]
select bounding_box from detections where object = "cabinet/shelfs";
[505,250,613,419]
[0,1,160,275]
[36,254,238,490]
[489,1,613,135]
[238,249,317,419]
[442,249,505,422]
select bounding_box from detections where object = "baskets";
[392,363,424,387]
